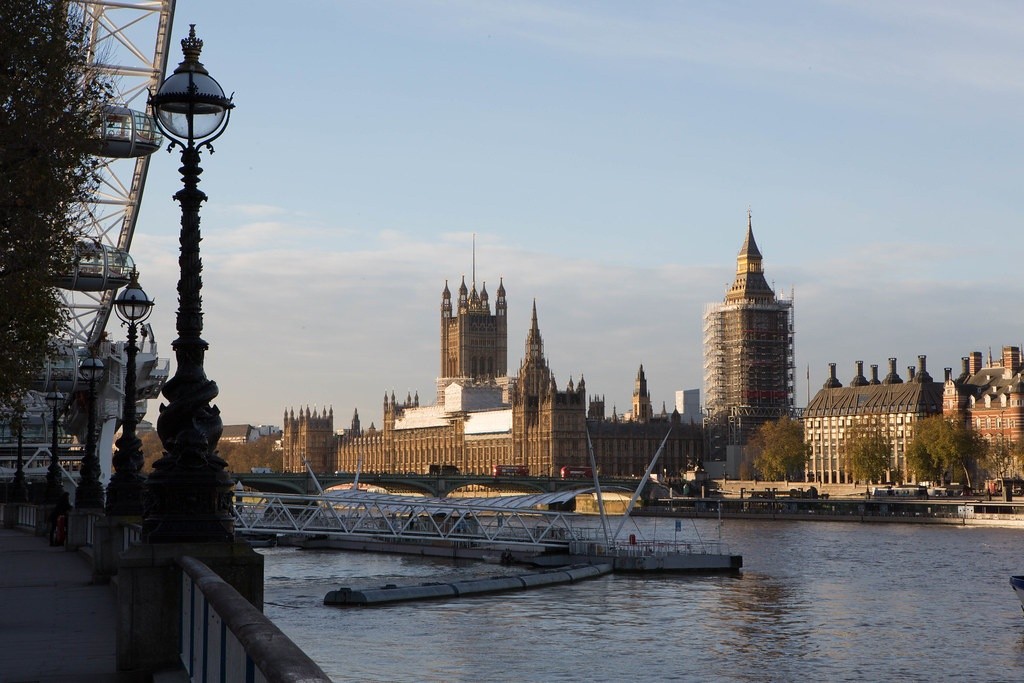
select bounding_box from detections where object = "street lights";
[45,380,65,498]
[103,263,156,518]
[11,411,30,502]
[137,23,237,541]
[76,347,105,509]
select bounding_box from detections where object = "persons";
[47,492,73,547]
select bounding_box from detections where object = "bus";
[492,465,529,477]
[561,466,593,480]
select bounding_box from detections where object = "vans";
[425,465,460,476]
